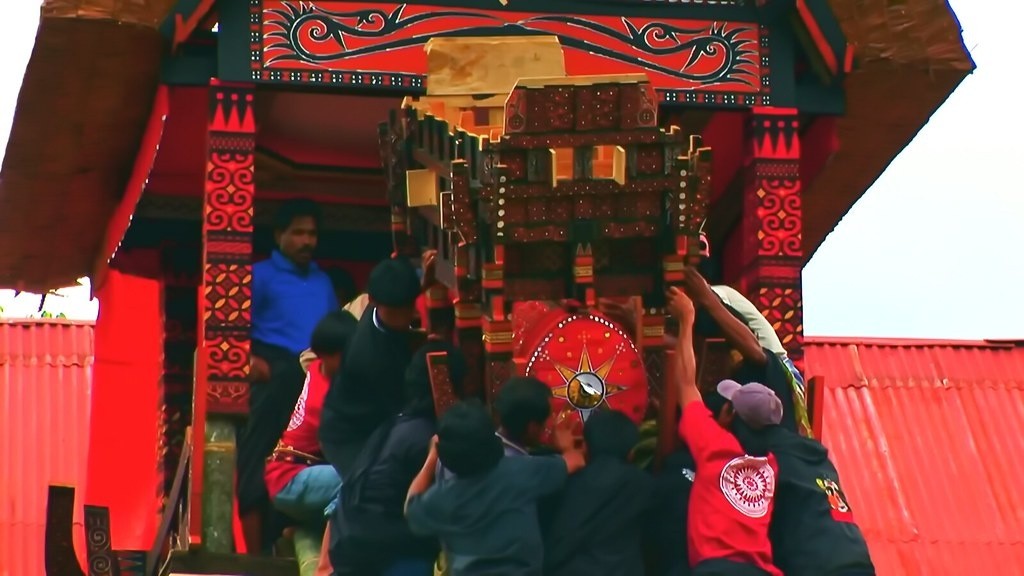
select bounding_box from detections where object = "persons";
[235,202,342,556]
[263,248,876,576]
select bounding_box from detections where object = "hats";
[716,379,783,428]
